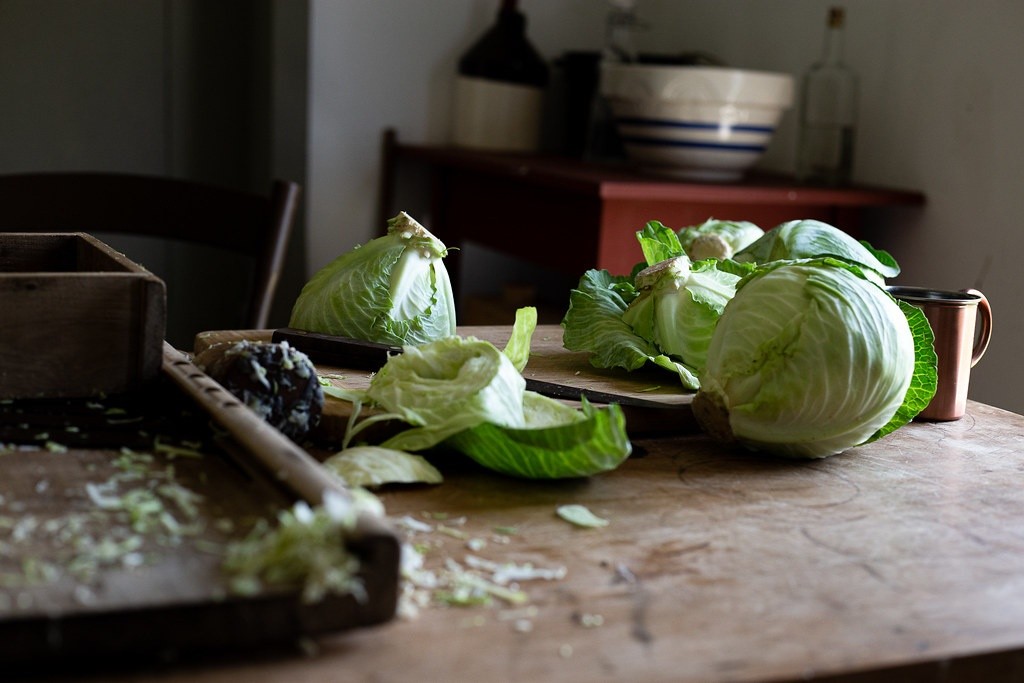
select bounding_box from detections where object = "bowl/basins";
[603,63,795,181]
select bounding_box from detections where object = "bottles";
[451,0,542,153]
[794,7,861,184]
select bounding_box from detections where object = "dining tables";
[3,306,1024,683]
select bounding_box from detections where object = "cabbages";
[322,298,633,489]
[561,216,935,459]
[287,216,457,348]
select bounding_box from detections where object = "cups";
[886,286,994,423]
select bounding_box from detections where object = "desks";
[371,127,928,310]
